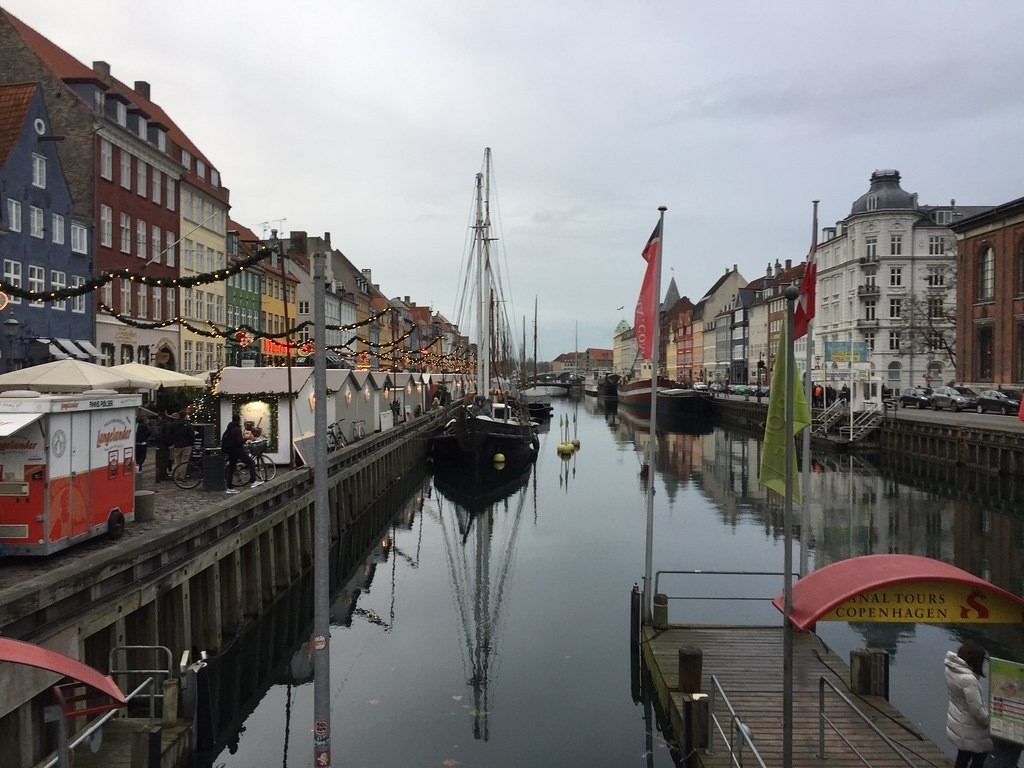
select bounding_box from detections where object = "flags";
[792,237,817,340]
[760,320,814,506]
[633,218,662,359]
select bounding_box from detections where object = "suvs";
[693,382,709,392]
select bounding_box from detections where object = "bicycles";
[326,418,349,454]
[352,419,366,441]
[172,435,277,489]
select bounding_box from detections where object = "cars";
[899,386,935,409]
[710,383,770,397]
[975,390,1022,415]
[930,386,980,413]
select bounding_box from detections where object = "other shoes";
[225,488,240,494]
[161,473,168,480]
[168,474,175,483]
[250,481,265,488]
[135,464,143,474]
[175,473,186,483]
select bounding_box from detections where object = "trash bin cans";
[678,646,702,692]
[201,448,227,492]
[851,648,870,697]
[653,593,669,628]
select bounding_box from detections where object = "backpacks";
[221,425,235,453]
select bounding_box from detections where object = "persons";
[137,417,152,473]
[944,639,994,768]
[708,380,711,387]
[224,414,265,494]
[154,409,195,482]
[812,382,836,407]
[839,384,848,405]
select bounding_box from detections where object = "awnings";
[34,336,73,359]
[0,413,46,436]
[77,341,110,358]
[56,338,89,358]
[327,356,342,363]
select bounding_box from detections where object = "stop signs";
[926,376,933,382]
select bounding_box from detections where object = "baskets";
[248,441,268,457]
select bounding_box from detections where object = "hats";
[183,415,191,420]
[168,412,180,419]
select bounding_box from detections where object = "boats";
[614,402,679,436]
[583,371,676,407]
[0,637,207,768]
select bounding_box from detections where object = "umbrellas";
[0,357,161,391]
[110,361,206,387]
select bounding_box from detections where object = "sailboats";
[427,146,586,465]
[428,453,538,740]
